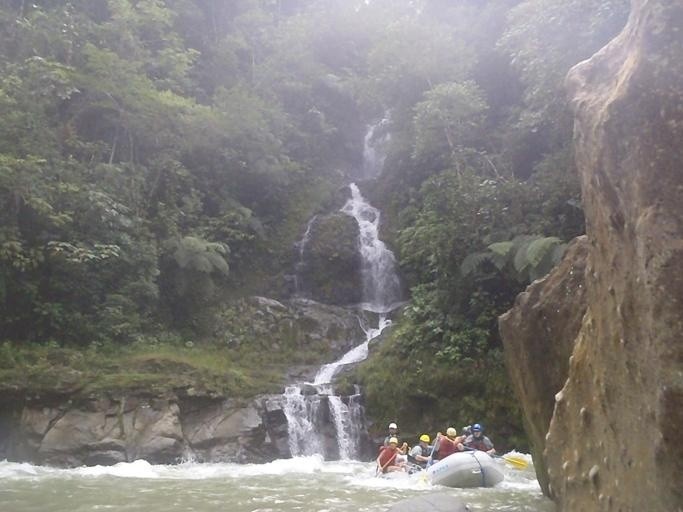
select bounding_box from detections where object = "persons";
[376,422,496,475]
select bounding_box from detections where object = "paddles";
[421,437,439,482]
[463,446,528,469]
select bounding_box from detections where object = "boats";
[372,449,506,488]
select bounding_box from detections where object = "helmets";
[420,435,430,442]
[447,427,456,437]
[388,423,397,429]
[390,437,398,444]
[467,423,482,432]
